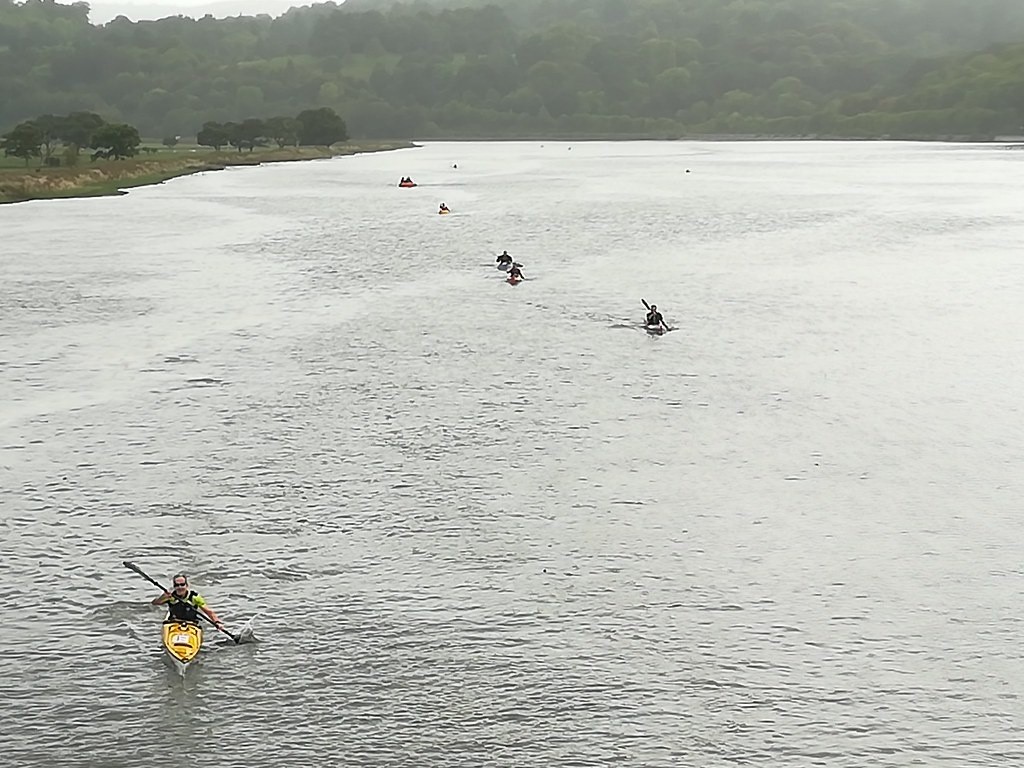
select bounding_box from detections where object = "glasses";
[176,583,185,586]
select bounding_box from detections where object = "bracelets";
[215,621,224,625]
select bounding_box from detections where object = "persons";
[400,163,664,326]
[151,575,224,630]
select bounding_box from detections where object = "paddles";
[122,561,236,638]
[641,298,668,330]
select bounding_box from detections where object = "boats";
[160,612,204,681]
[646,323,665,335]
[498,261,522,285]
